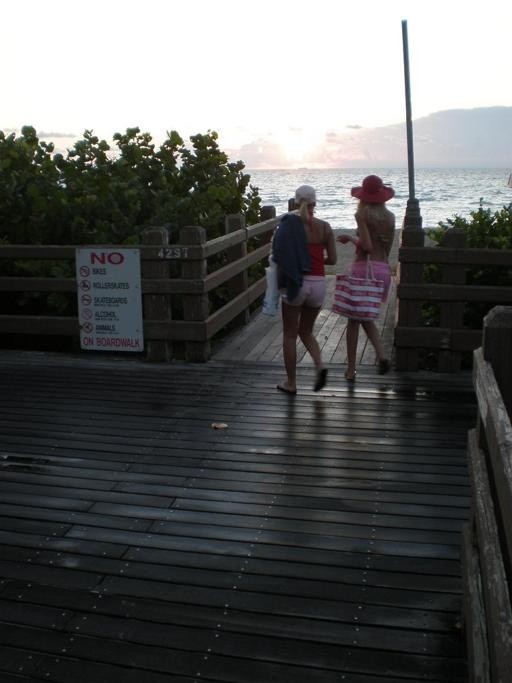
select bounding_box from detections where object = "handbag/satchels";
[262,266,280,316]
[332,274,384,321]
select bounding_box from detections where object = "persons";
[335,176,396,381]
[272,186,337,395]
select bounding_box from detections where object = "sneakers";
[344,369,357,381]
[379,359,389,376]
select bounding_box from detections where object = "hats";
[351,175,395,203]
[295,185,316,204]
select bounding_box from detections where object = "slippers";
[277,380,297,393]
[313,368,328,391]
